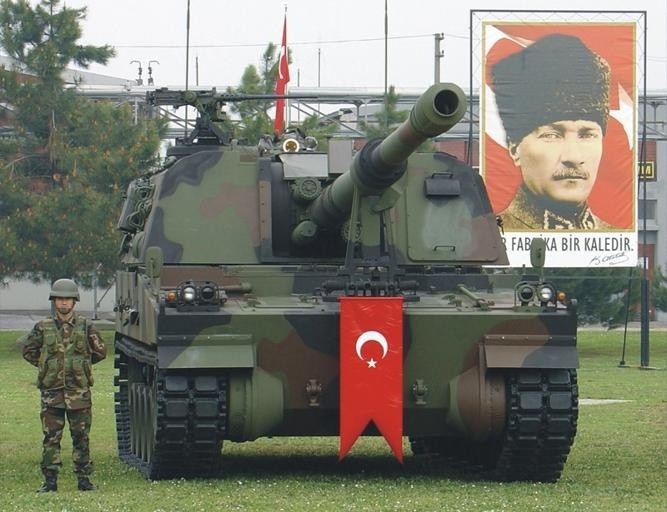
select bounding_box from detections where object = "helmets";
[49,278,81,302]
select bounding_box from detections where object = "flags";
[272,4,292,141]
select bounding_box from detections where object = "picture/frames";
[481,20,636,233]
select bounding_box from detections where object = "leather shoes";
[78,476,97,490]
[36,474,58,492]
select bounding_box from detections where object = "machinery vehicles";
[114,89,579,482]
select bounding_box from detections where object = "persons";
[21,277,107,494]
[491,30,620,235]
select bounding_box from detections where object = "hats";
[490,33,611,145]
